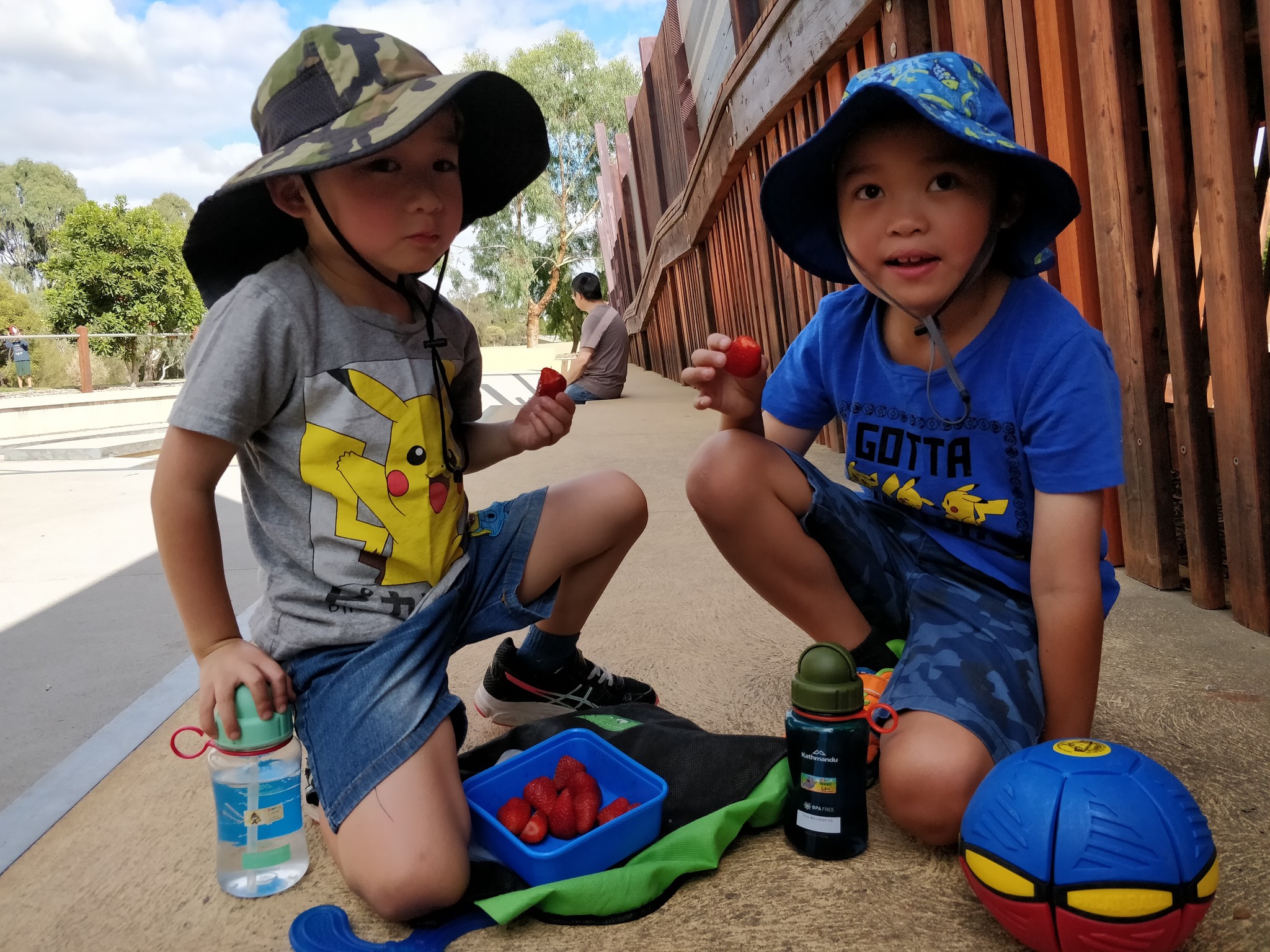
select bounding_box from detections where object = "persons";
[688,50,1126,842]
[566,273,630,404]
[151,26,664,919]
[5,329,33,389]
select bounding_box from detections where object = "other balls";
[957,737,1219,952]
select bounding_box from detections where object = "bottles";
[784,642,900,861]
[169,683,311,899]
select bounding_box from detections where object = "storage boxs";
[462,726,668,883]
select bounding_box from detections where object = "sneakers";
[855,639,909,774]
[304,757,321,823]
[474,636,658,726]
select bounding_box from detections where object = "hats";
[761,51,1081,289]
[181,25,548,309]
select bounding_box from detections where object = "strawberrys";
[724,335,762,379]
[497,754,642,843]
[538,367,567,399]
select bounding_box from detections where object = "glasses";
[570,292,580,300]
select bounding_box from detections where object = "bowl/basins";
[461,728,668,886]
